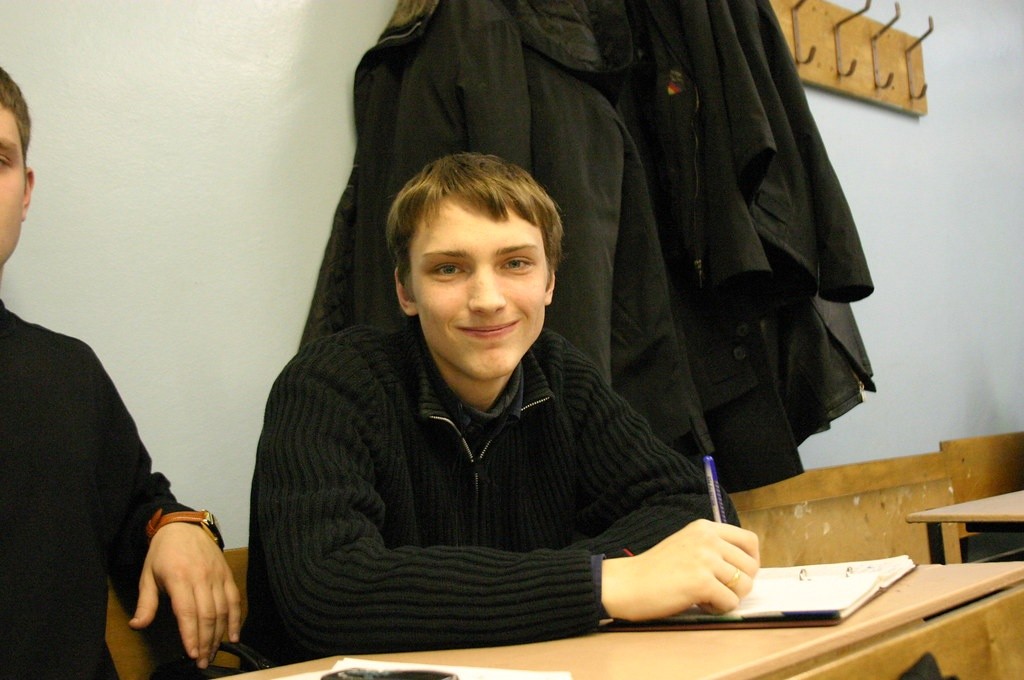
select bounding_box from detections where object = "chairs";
[727,432,1024,564]
[106,547,248,679]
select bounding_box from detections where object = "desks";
[906,489,1024,565]
[216,561,1024,680]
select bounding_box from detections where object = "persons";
[0,67,244,680]
[246,153,764,660]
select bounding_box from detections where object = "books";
[602,556,916,625]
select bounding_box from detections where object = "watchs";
[146,507,225,553]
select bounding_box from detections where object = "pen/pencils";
[702,455,726,523]
